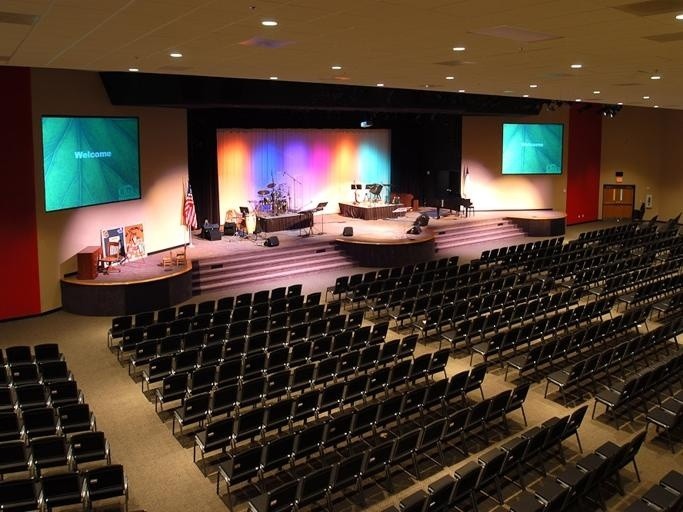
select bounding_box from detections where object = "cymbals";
[258,190,269,195]
[267,183,276,188]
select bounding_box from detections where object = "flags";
[184,182,197,230]
[463,167,471,199]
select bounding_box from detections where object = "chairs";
[0,204,683,512]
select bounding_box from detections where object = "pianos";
[437,195,470,219]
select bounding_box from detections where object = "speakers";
[201,224,219,238]
[206,230,221,241]
[343,227,353,236]
[224,222,236,235]
[413,214,429,226]
[264,236,279,247]
[407,226,421,234]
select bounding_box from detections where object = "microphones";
[283,172,285,176]
